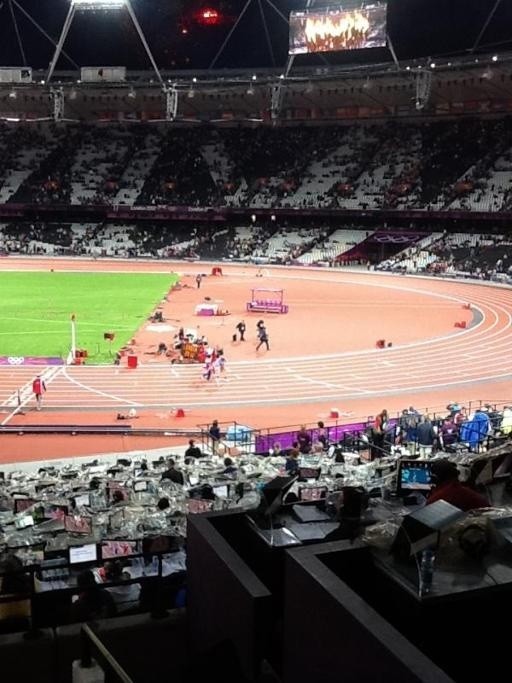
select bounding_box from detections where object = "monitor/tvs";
[0,445,380,572]
[396,459,438,494]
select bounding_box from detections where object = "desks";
[1,412,512,683]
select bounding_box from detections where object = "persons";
[256,320,270,351]
[236,320,245,341]
[6,440,260,625]
[212,401,511,541]
[33,375,47,409]
[196,274,201,288]
[1,118,510,280]
[159,327,225,380]
